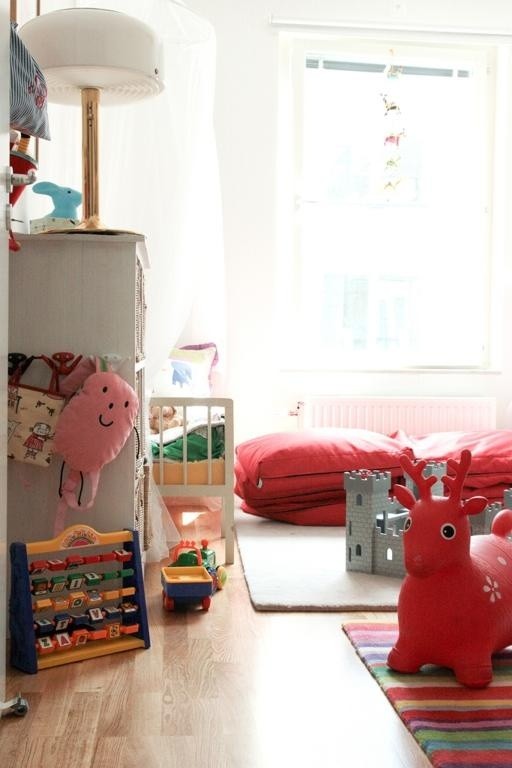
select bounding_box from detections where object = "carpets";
[229,491,416,615]
[341,615,511,765]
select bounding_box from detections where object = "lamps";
[10,1,171,232]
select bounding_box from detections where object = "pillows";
[165,341,220,400]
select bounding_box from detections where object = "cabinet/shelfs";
[7,223,151,627]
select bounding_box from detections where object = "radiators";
[310,391,499,446]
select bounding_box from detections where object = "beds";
[145,338,240,567]
